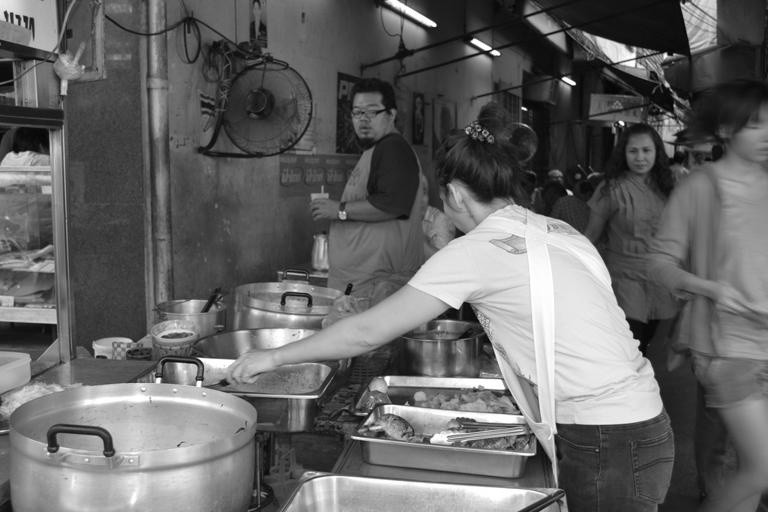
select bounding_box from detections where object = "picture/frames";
[432,99,458,158]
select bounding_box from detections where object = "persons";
[541,181,591,236]
[668,151,689,187]
[548,169,573,196]
[689,153,705,172]
[584,122,674,357]
[308,77,422,384]
[514,170,546,215]
[421,174,456,259]
[225,100,675,512]
[643,82,768,512]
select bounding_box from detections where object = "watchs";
[337,202,348,222]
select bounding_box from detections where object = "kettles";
[311,230,331,271]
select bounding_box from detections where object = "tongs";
[429,418,533,447]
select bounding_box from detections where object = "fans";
[197,52,313,158]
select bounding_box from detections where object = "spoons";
[350,411,416,445]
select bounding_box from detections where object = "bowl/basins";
[93,336,133,354]
[402,319,485,378]
[193,329,352,370]
[1,351,30,396]
[150,321,199,346]
[126,361,337,433]
[279,469,566,512]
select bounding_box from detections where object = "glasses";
[350,106,391,120]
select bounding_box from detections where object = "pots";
[234,268,352,328]
[153,299,226,340]
[10,355,257,512]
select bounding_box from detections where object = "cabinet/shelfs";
[0,39,76,378]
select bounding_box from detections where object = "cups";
[311,193,329,218]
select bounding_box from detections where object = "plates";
[349,402,538,479]
[352,376,526,417]
